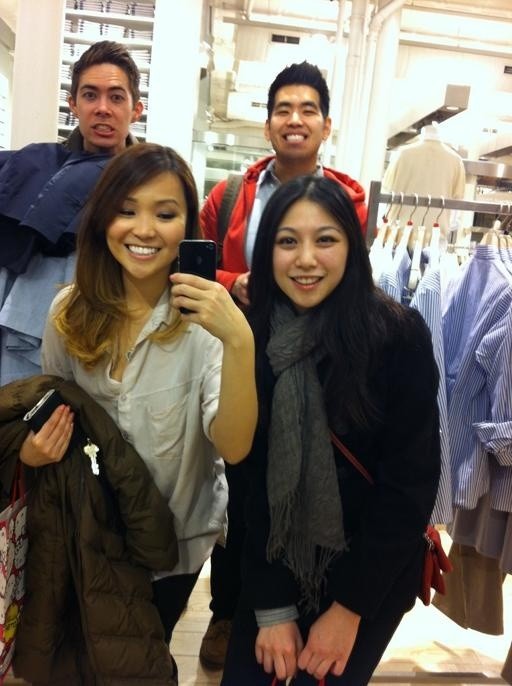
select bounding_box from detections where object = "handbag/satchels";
[417,522,453,606]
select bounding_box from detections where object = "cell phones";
[179,240,216,314]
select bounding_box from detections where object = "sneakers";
[199,609,233,671]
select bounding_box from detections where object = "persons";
[2,39,145,403]
[376,123,468,231]
[196,59,377,671]
[216,172,444,686]
[2,140,260,686]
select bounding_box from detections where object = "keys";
[84,436,101,477]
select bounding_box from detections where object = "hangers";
[378,192,512,290]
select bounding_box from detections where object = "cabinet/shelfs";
[57,1,156,150]
